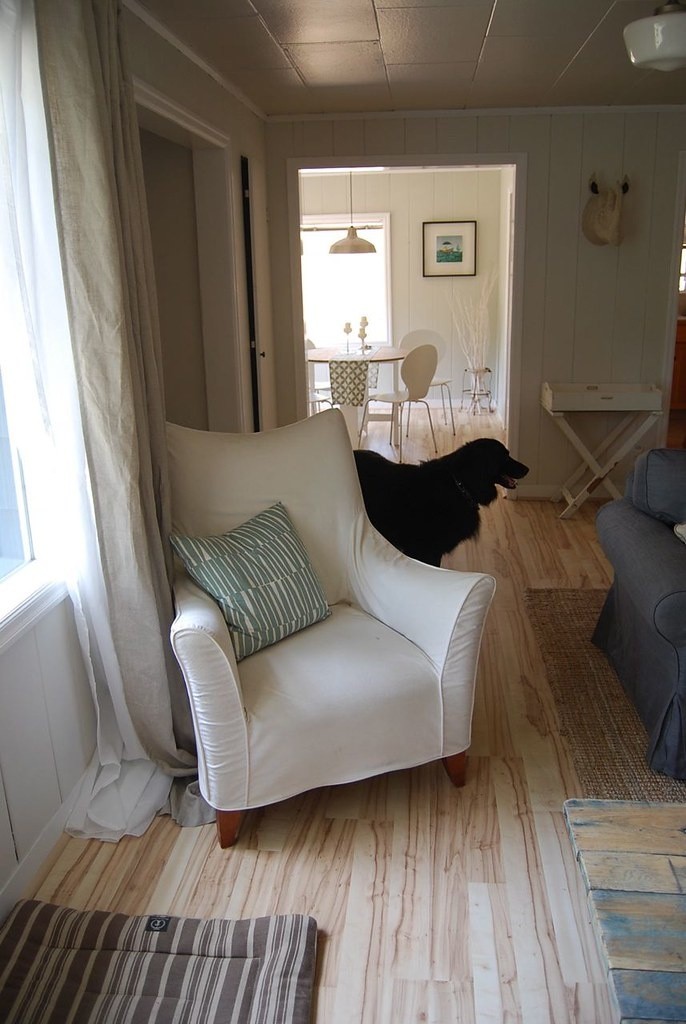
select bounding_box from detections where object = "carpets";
[523,586,686,803]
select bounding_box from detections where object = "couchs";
[590,447,686,781]
[164,407,495,851]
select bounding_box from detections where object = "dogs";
[353,438,530,567]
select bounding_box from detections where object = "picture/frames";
[422,221,477,277]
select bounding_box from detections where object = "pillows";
[170,500,333,662]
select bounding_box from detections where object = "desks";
[306,345,406,450]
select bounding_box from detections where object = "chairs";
[400,329,457,437]
[358,344,439,463]
[305,338,333,418]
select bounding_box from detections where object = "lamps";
[622,0,686,73]
[328,172,377,254]
